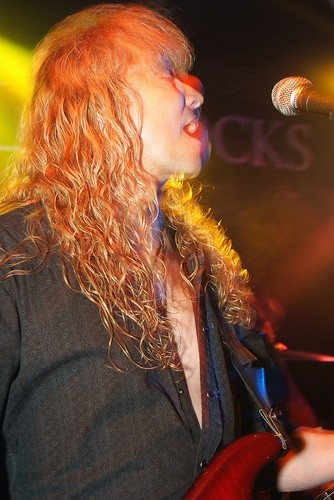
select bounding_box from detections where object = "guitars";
[183,432,334,500]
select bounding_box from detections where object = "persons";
[1,3,295,500]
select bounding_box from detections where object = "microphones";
[271,76,333,118]
